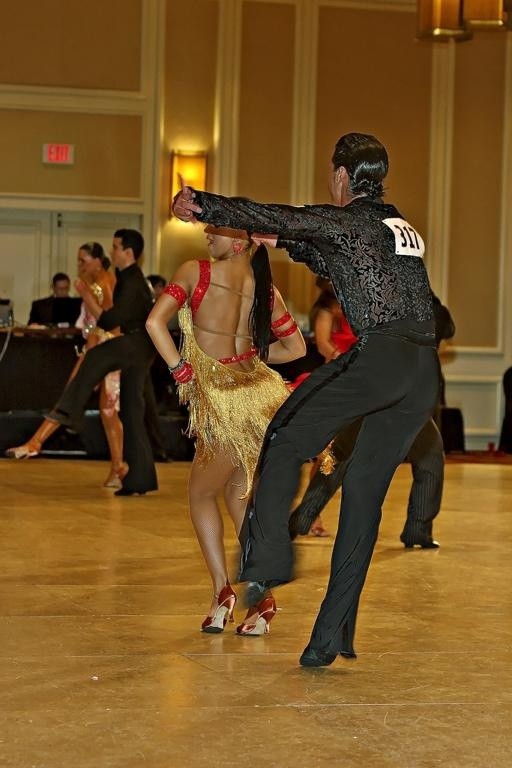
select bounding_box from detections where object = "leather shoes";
[115,483,157,496]
[299,644,337,665]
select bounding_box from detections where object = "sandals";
[310,526,329,537]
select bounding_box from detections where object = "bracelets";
[168,358,193,384]
[327,348,339,361]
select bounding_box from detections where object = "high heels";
[237,590,277,636]
[5,444,40,463]
[201,583,236,632]
[104,461,129,486]
[405,540,440,548]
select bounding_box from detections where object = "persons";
[172,132,443,667]
[6,229,165,496]
[289,292,456,550]
[285,277,359,537]
[144,223,307,636]
